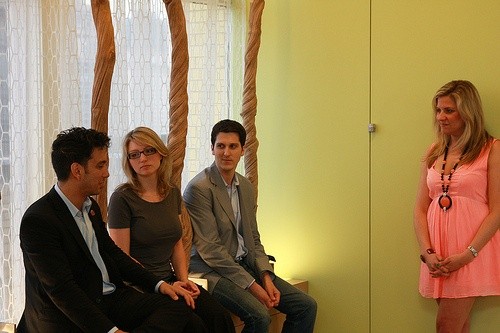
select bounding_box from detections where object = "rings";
[277,303,279,306]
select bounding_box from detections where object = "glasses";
[126,145,157,161]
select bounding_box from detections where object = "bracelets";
[418,248,436,264]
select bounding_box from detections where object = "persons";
[107,126,237,333]
[413,78,500,333]
[180,119,318,333]
[19,127,195,333]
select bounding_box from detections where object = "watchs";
[466,245,478,257]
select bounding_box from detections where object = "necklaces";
[438,133,475,212]
